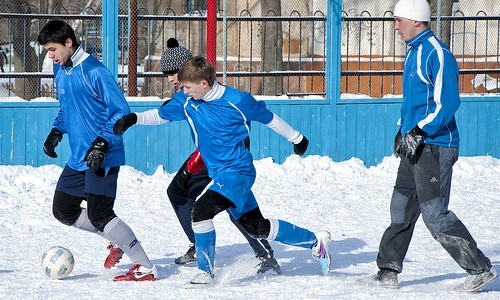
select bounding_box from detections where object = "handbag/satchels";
[394,128,404,158]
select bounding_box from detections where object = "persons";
[0,46,9,73]
[37,19,158,282]
[357,0,497,295]
[160,37,281,278]
[113,57,332,288]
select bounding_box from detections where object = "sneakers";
[113,263,158,282]
[253,258,281,276]
[103,242,125,270]
[175,243,197,265]
[189,270,212,284]
[310,230,331,276]
[356,267,399,289]
[453,265,498,292]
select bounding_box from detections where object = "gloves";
[83,136,109,173]
[113,113,137,135]
[43,128,63,158]
[402,125,427,165]
[175,167,191,192]
[293,135,308,156]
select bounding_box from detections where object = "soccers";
[41,246,74,280]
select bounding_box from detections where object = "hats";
[160,38,192,75]
[393,0,431,21]
